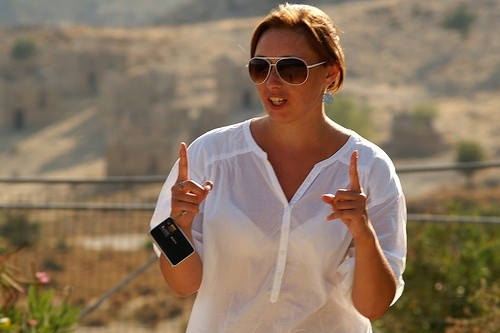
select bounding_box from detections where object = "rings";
[177,182,184,190]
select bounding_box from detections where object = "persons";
[147,0,409,333]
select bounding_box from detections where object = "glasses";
[244,57,327,87]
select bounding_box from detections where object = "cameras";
[148,217,196,267]
